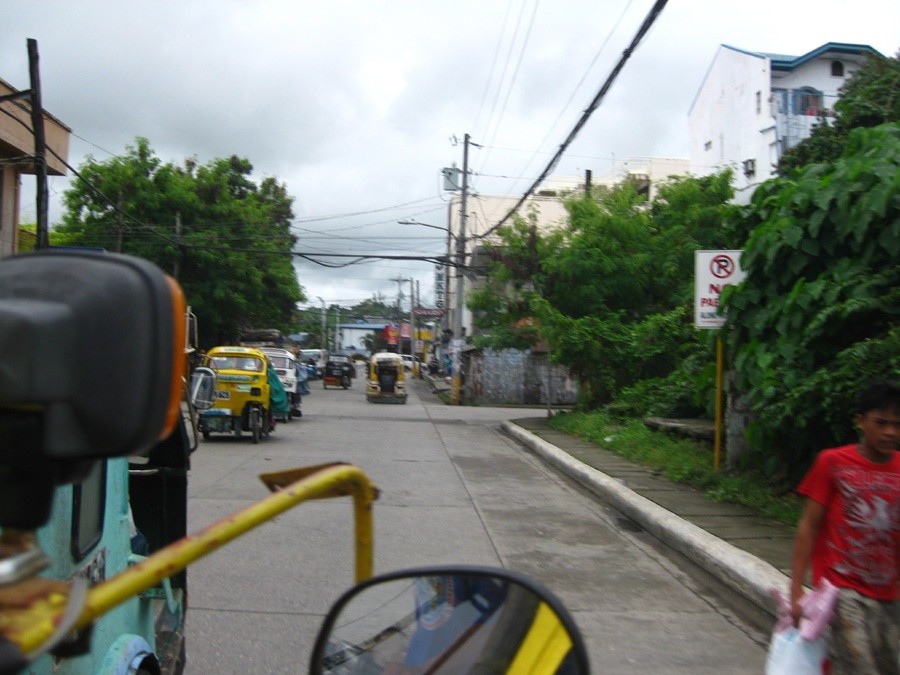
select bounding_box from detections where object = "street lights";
[389,278,416,377]
[397,221,460,406]
[317,296,326,349]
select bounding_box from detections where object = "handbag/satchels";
[763,625,831,675]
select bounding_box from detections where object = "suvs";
[400,355,428,373]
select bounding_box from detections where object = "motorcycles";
[367,352,408,404]
[196,346,275,444]
[258,347,303,424]
[0,246,596,675]
[298,349,328,381]
[323,353,357,390]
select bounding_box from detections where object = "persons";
[293,344,310,394]
[790,383,900,675]
[427,354,439,374]
[308,357,318,379]
[437,354,452,374]
